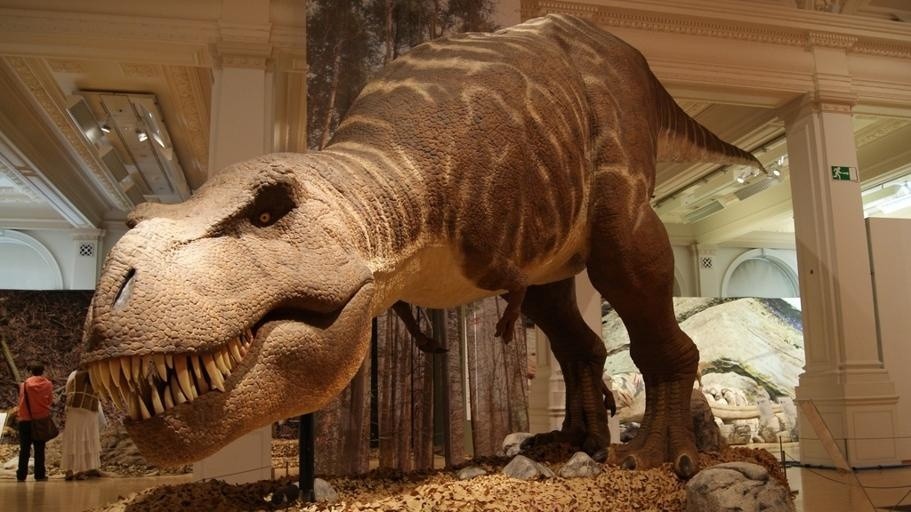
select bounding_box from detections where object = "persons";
[17,361,53,481]
[63,368,108,480]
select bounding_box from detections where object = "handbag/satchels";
[25,417,60,446]
